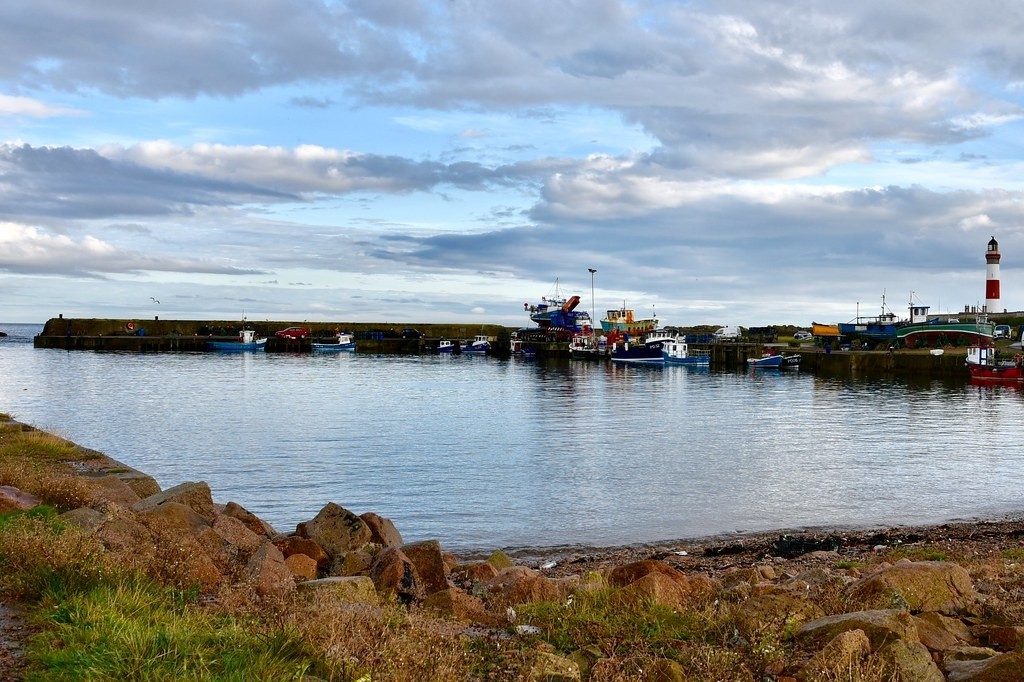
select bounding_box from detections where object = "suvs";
[793,333,812,340]
[400,328,425,339]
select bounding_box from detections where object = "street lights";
[588,268,597,342]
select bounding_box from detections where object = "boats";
[966,338,1024,381]
[747,348,802,368]
[311,336,357,354]
[811,288,993,349]
[512,277,711,365]
[437,335,491,354]
[202,330,268,351]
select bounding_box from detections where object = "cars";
[993,325,1013,341]
[275,327,310,340]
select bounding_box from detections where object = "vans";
[715,328,742,343]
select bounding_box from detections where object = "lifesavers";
[593,341,599,349]
[1014,353,1023,367]
